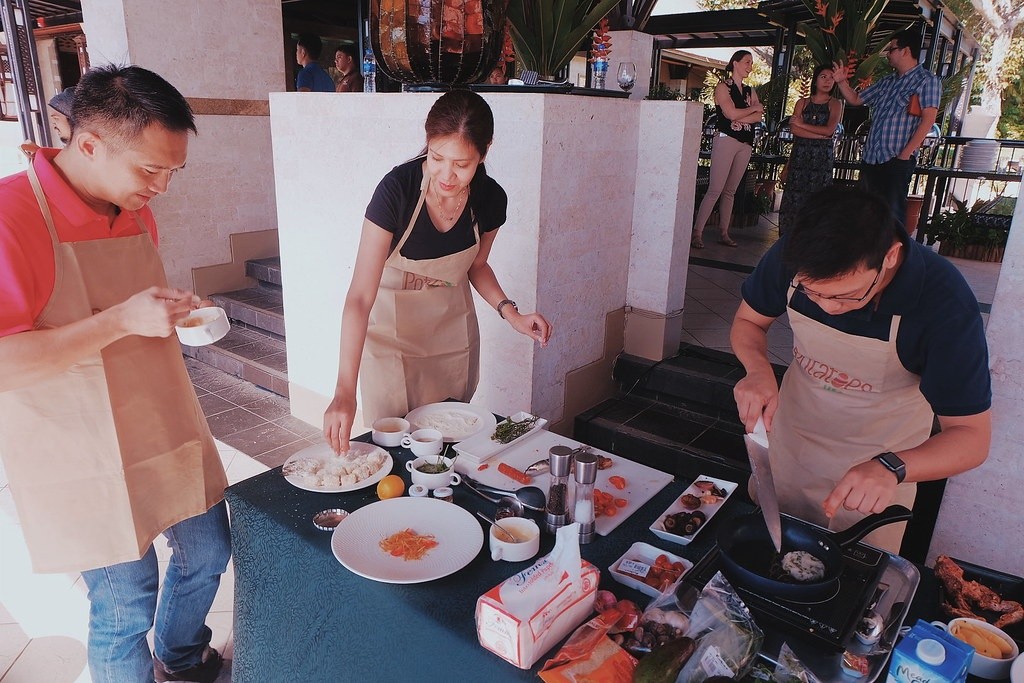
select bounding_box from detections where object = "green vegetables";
[491,414,540,443]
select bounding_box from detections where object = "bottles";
[364,49,377,93]
[572,451,598,544]
[544,445,573,535]
[591,44,608,89]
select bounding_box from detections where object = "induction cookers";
[683,504,891,653]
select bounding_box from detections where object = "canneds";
[409,484,428,497]
[433,487,453,504]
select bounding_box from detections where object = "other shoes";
[717,235,737,247]
[691,236,704,248]
[152,644,223,683]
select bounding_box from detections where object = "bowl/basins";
[405,454,462,490]
[174,306,231,347]
[489,516,540,563]
[372,417,410,447]
[401,428,443,458]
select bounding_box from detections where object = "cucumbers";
[631,636,696,683]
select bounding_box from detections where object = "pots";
[717,504,913,601]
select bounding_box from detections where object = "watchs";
[497,299,519,319]
[871,451,906,485]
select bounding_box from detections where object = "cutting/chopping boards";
[450,429,675,536]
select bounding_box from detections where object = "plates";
[404,401,497,443]
[452,411,548,464]
[608,541,695,599]
[959,140,1001,172]
[648,474,739,546]
[282,440,393,493]
[330,495,484,585]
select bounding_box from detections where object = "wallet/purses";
[908,94,923,116]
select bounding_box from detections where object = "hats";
[48,86,76,119]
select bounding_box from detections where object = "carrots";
[379,529,438,561]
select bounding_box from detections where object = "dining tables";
[224,397,932,683]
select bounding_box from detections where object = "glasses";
[791,259,884,304]
[889,47,897,54]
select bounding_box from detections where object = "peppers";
[608,476,625,489]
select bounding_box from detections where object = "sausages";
[497,463,530,485]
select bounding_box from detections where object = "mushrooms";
[611,620,682,659]
[680,493,702,511]
[663,510,706,537]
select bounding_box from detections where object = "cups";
[930,617,1020,681]
[617,61,637,91]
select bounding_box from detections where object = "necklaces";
[431,180,467,221]
[811,97,830,120]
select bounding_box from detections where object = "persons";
[324,89,554,458]
[729,183,992,564]
[295,31,336,92]
[691,50,764,248]
[18,86,78,161]
[334,45,365,92]
[489,66,508,85]
[0,61,233,683]
[833,30,942,225]
[779,63,842,239]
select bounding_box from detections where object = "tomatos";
[617,555,686,593]
[594,488,628,516]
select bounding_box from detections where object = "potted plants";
[931,195,1009,261]
[694,185,772,228]
[874,60,975,238]
[750,70,795,205]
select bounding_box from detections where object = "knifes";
[743,410,781,554]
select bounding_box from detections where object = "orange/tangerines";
[376,475,404,500]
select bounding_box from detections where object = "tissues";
[474,520,601,671]
